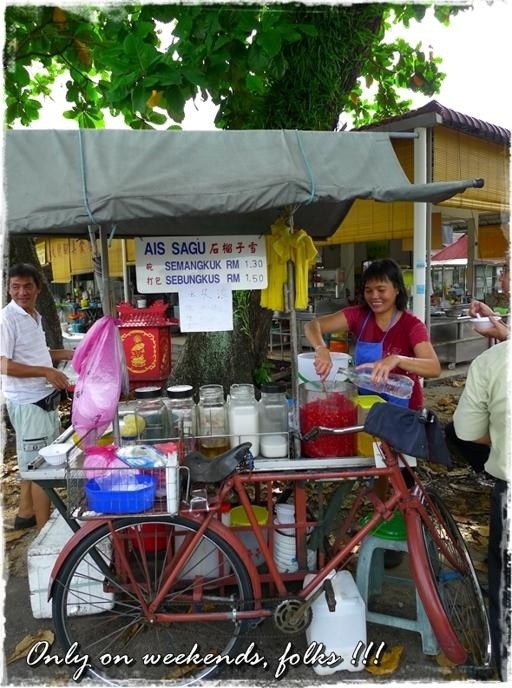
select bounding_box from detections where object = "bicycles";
[48,408,495,686]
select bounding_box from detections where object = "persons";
[450,337,510,679]
[302,257,441,572]
[468,249,510,345]
[3,260,76,533]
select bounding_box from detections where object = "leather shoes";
[14,513,37,530]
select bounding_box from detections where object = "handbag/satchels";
[30,389,61,412]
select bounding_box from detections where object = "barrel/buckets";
[301,568,369,678]
[297,352,353,383]
[273,520,318,575]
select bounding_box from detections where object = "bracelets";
[314,344,327,351]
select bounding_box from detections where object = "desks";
[78,307,101,320]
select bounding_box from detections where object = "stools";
[356,511,445,656]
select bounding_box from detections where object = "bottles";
[337,364,414,401]
[122,382,290,458]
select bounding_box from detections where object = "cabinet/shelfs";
[309,266,346,299]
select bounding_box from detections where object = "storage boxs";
[27,508,113,619]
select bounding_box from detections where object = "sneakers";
[384,548,404,569]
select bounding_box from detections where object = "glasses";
[500,269,509,277]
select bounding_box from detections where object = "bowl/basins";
[495,307,507,314]
[273,501,297,535]
[471,316,502,331]
[298,351,351,388]
[38,444,70,465]
[445,306,470,317]
